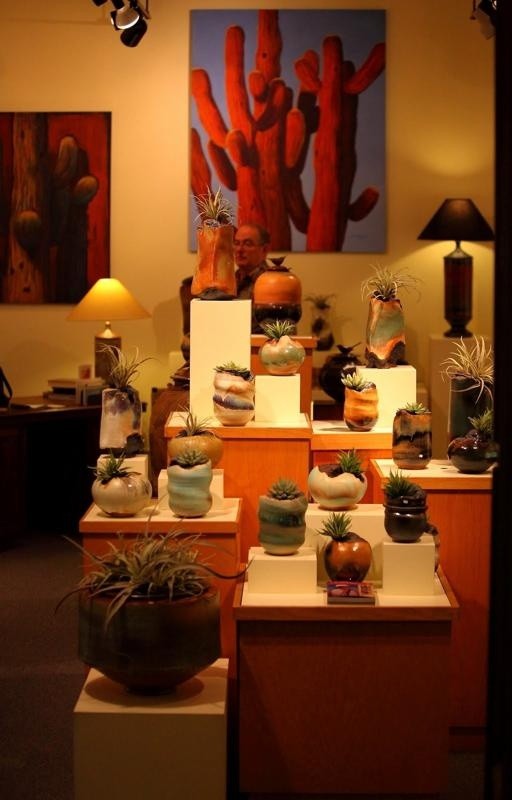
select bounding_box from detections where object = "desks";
[0,394,147,551]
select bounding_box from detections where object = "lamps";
[92,0,151,48]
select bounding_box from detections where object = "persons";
[231,224,272,301]
